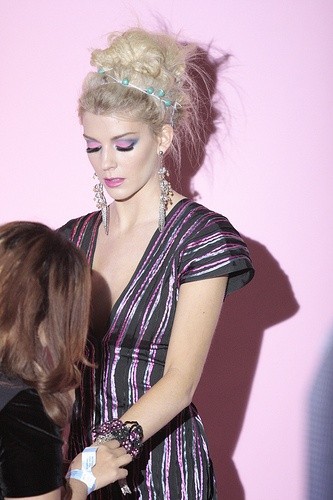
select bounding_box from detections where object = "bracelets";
[91,418,144,457]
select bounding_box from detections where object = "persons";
[39,24,257,500]
[0,220,134,500]
